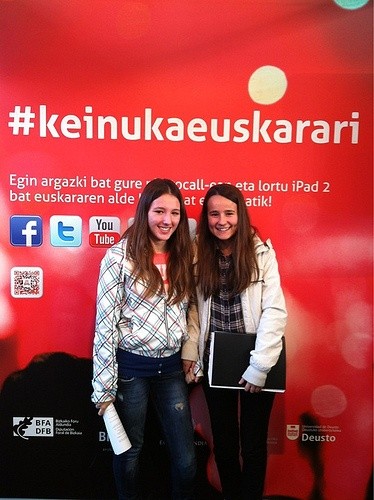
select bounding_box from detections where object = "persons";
[91,178,207,500]
[180,183,288,499]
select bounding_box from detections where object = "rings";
[96,404,101,408]
